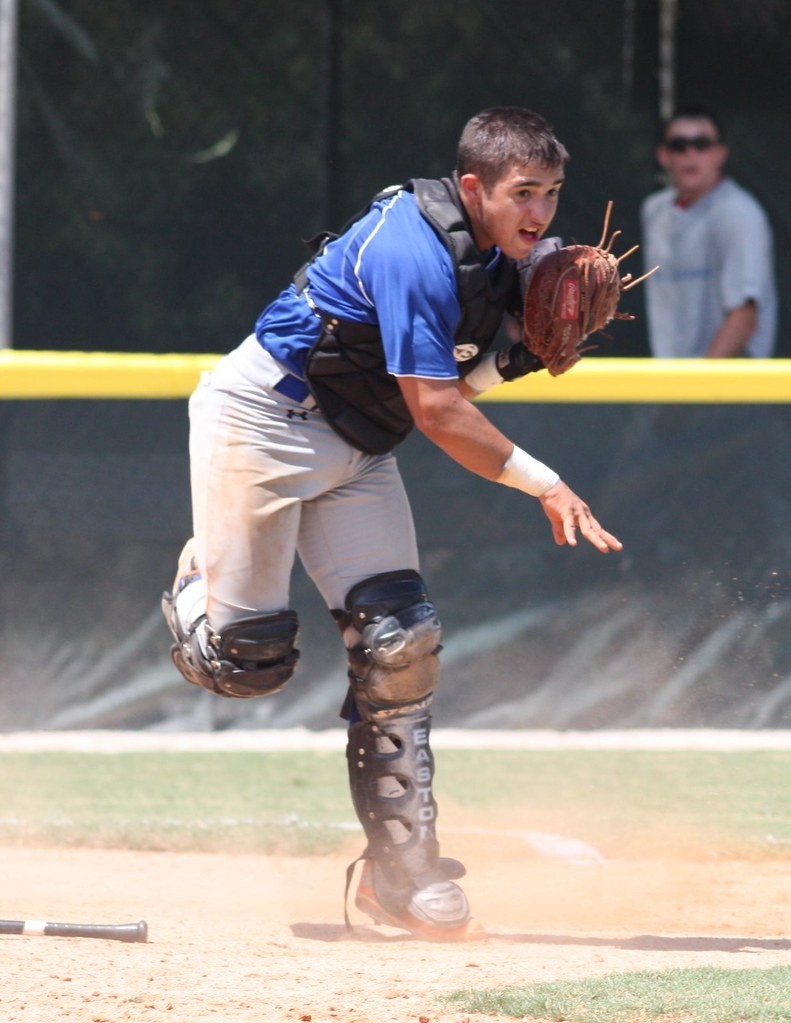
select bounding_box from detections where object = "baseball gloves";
[519,237,625,372]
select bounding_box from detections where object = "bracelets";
[495,444,561,499]
[464,350,503,393]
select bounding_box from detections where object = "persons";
[636,110,781,606]
[158,105,658,940]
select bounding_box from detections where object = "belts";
[275,375,319,411]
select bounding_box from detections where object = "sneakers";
[346,860,487,944]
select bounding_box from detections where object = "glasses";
[665,135,718,152]
